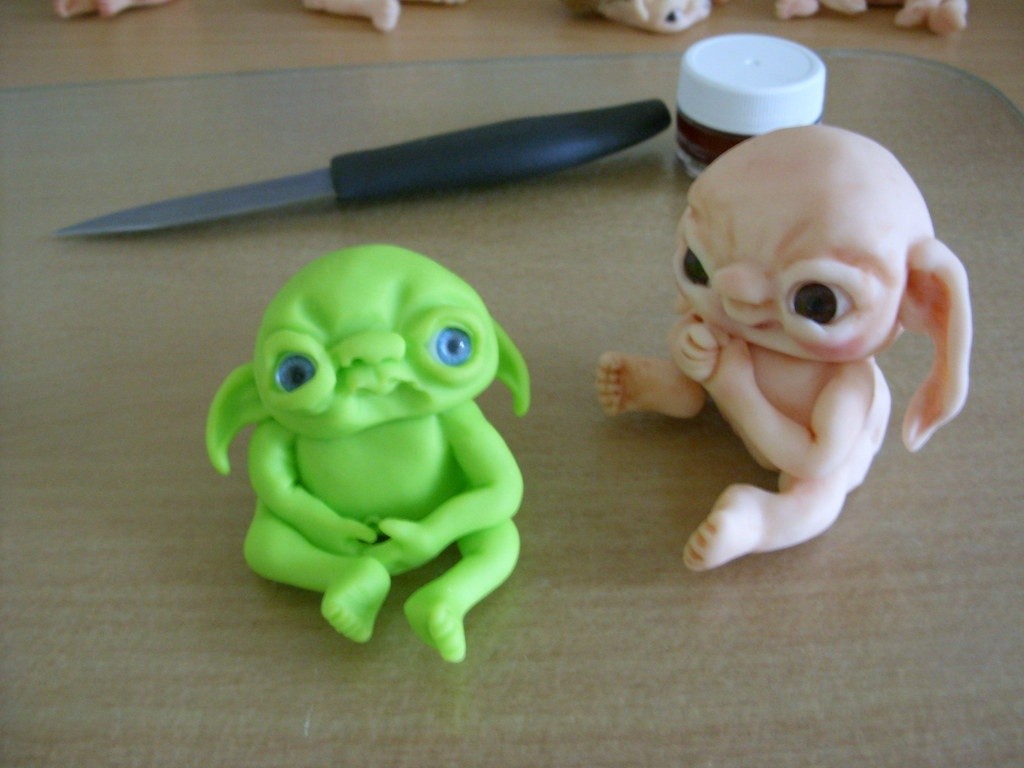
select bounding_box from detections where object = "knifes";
[52,99,671,236]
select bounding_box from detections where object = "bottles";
[677,34,826,176]
